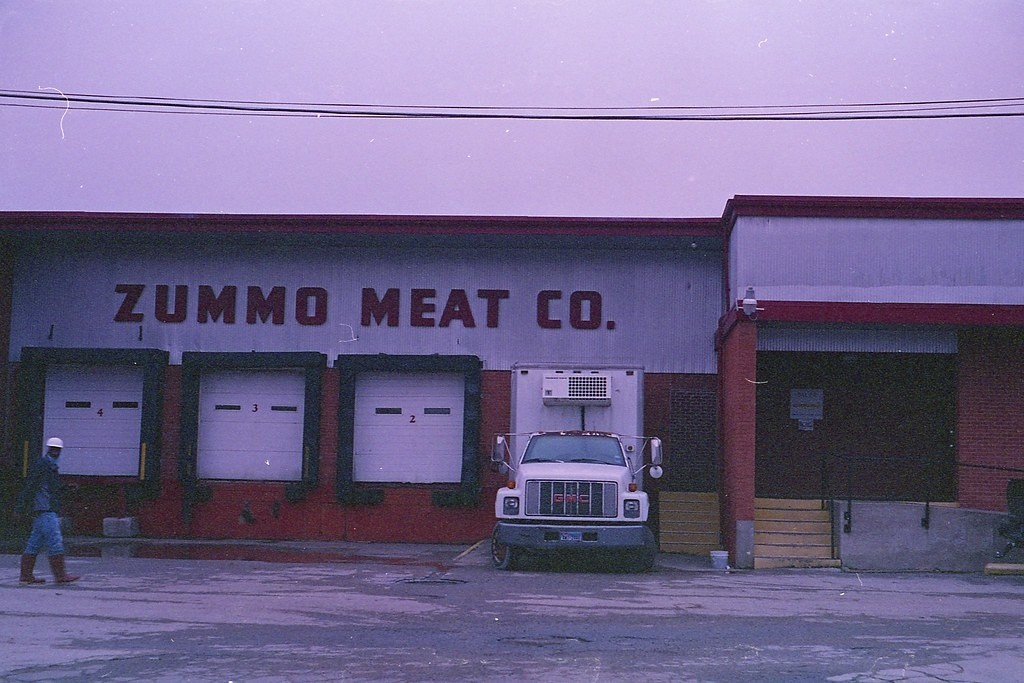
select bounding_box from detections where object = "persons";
[14,438,81,584]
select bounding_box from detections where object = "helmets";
[47,438,64,449]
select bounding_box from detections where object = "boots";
[49,554,80,583]
[18,553,46,585]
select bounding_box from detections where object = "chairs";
[995,479,1024,558]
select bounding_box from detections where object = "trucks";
[488,360,663,571]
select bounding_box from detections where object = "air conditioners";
[540,375,612,407]
